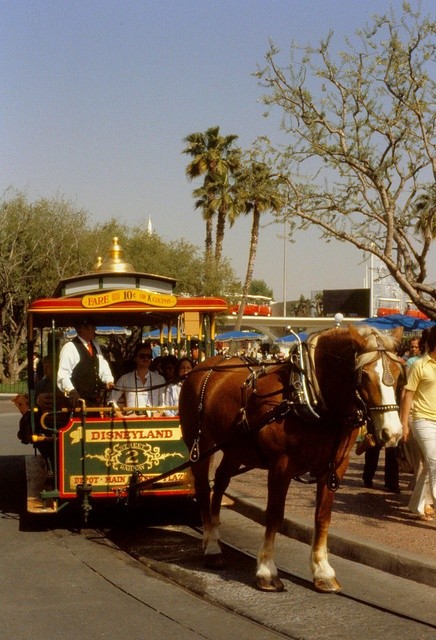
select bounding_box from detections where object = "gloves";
[69,390,78,408]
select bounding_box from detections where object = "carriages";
[25,271,404,592]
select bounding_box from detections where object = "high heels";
[409,510,433,521]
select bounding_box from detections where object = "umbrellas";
[275,333,311,346]
[142,327,204,338]
[65,327,132,338]
[352,314,435,331]
[214,331,268,353]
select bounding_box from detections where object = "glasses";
[136,353,152,361]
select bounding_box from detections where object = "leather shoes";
[364,479,373,487]
[384,486,400,493]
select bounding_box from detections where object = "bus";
[226,293,271,316]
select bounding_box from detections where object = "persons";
[168,359,195,417]
[57,316,115,407]
[404,330,422,380]
[402,325,436,519]
[108,344,167,415]
[191,340,207,366]
[32,356,54,393]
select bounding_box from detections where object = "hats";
[191,340,205,350]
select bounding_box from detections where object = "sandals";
[425,507,434,514]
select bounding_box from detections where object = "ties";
[86,343,94,356]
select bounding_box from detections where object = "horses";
[178,324,405,593]
